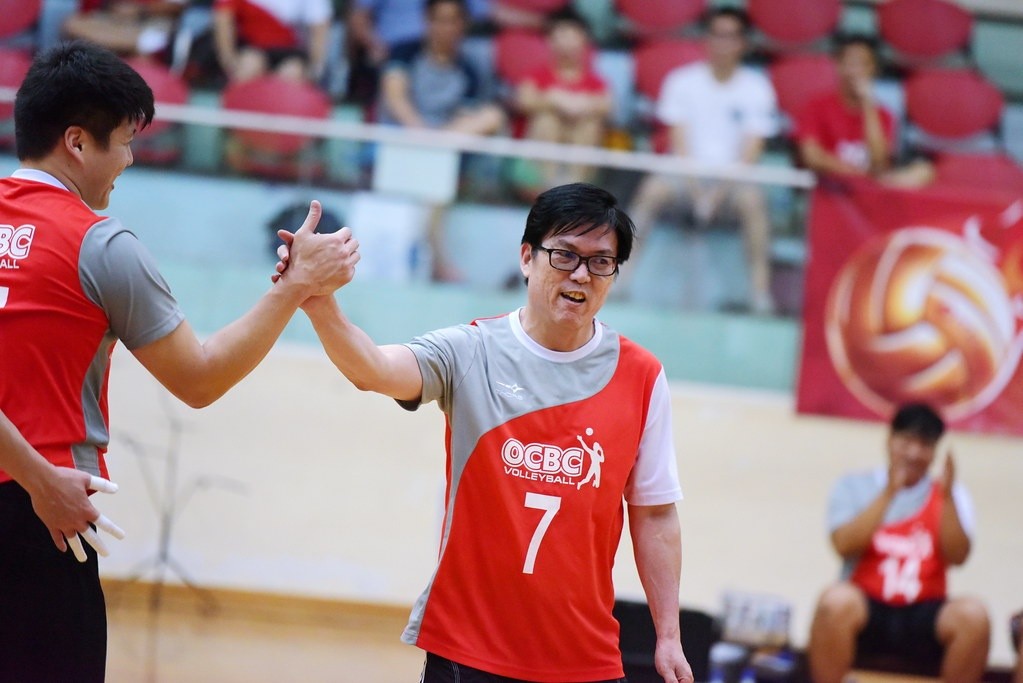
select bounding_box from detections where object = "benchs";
[0,0,1023,329]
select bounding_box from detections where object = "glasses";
[534,242,621,277]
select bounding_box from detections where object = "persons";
[631,8,784,314]
[808,399,994,683]
[0,41,361,683]
[269,182,696,683]
[0,0,1023,683]
